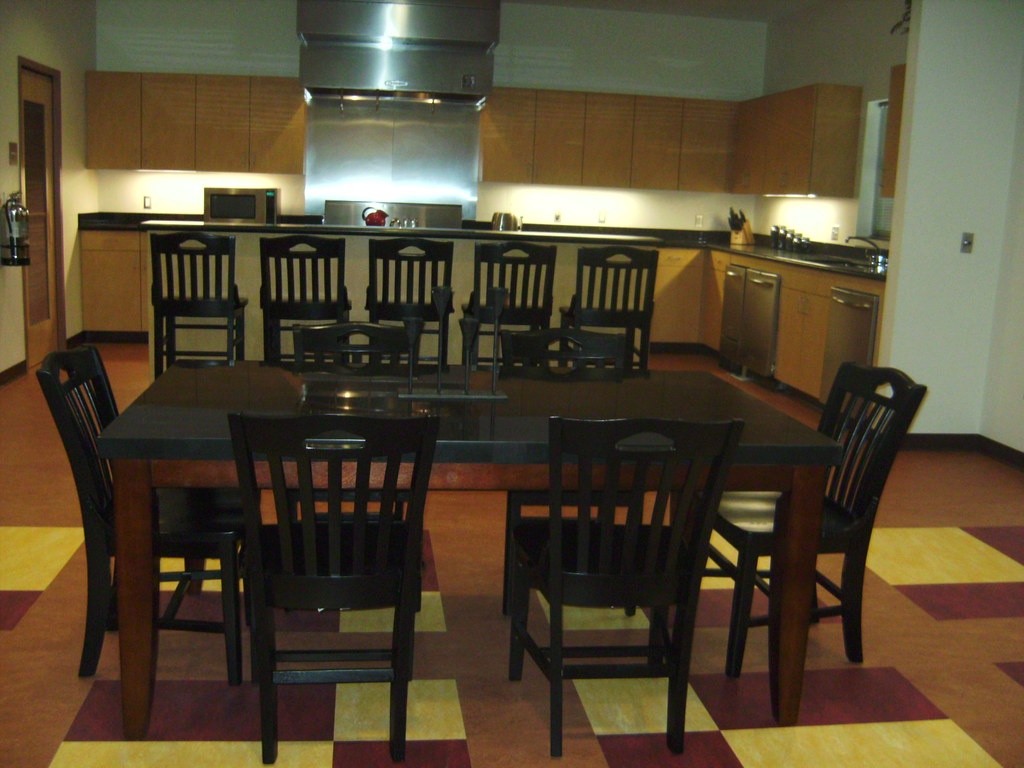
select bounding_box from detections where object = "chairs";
[33,230,747,765]
[696,360,928,675]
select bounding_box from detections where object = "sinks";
[802,258,867,269]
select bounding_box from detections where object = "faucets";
[844,235,884,267]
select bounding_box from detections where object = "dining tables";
[96,358,845,717]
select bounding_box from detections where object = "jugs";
[361,207,389,226]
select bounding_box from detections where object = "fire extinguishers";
[0,192,30,265]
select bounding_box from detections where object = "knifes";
[726,206,747,233]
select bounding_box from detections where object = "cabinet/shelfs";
[78,69,917,436]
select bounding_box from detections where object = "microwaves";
[202,186,281,228]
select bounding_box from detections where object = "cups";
[390,217,420,229]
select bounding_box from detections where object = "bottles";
[770,225,812,253]
